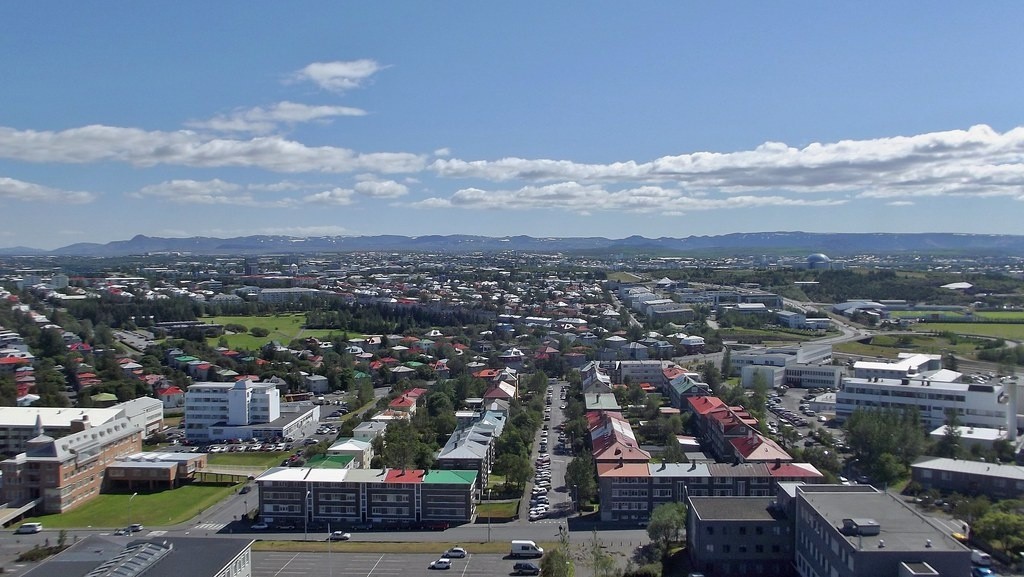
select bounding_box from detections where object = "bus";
[971,548,993,567]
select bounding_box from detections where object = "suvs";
[513,562,542,576]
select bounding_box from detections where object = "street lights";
[127,492,138,535]
[304,491,311,541]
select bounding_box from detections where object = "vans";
[511,539,544,558]
[15,522,43,534]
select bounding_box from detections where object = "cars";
[973,565,993,577]
[113,529,126,535]
[125,524,144,532]
[445,547,467,558]
[529,384,569,521]
[406,520,451,532]
[165,389,349,468]
[329,530,351,540]
[916,491,973,542]
[962,368,1020,388]
[351,521,374,533]
[428,558,452,569]
[275,521,296,532]
[749,383,874,484]
[250,521,269,530]
